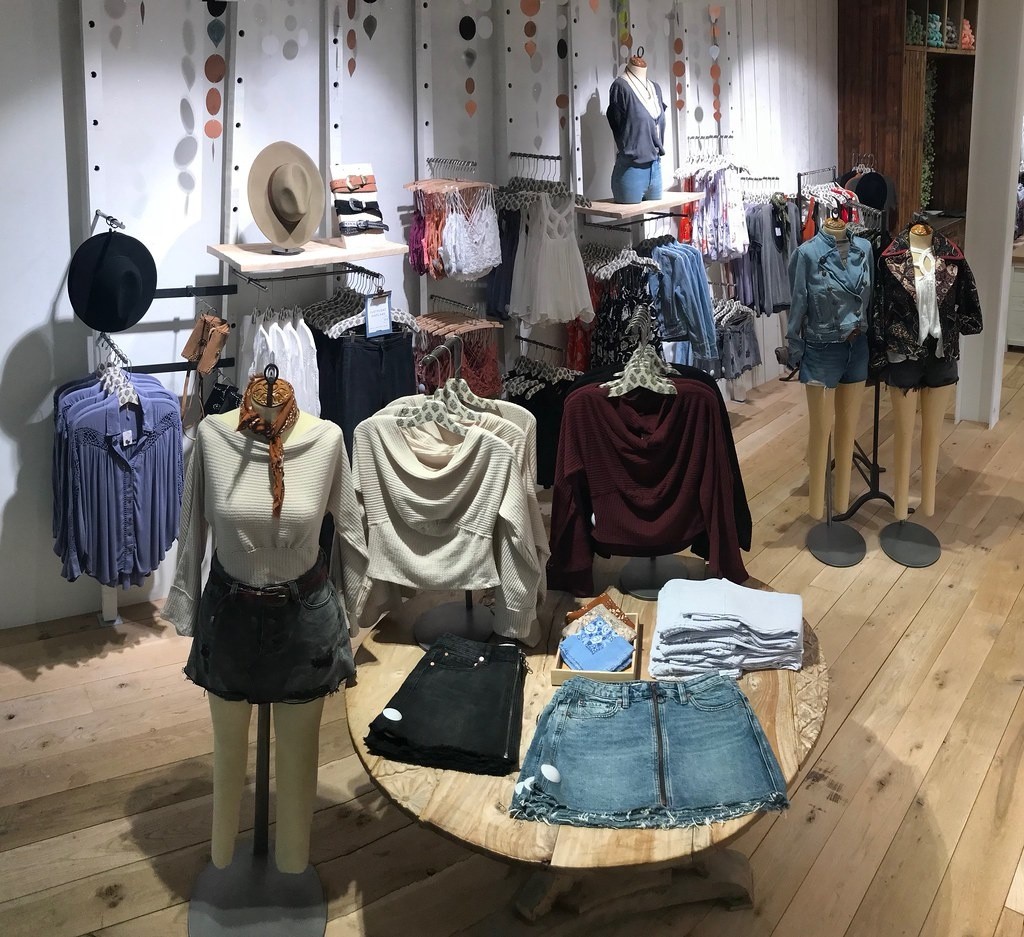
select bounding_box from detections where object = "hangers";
[243,131,879,465]
[52,331,182,427]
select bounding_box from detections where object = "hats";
[247,140,326,250]
[67,232,158,333]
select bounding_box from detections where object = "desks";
[343,540,831,925]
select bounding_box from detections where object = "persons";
[159,378,368,874]
[871,222,983,518]
[786,220,876,518]
[607,57,666,204]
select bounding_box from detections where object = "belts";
[339,220,389,235]
[330,174,377,193]
[334,197,382,218]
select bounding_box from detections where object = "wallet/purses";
[181,314,228,363]
[200,383,239,421]
[218,390,243,414]
[197,323,230,376]
